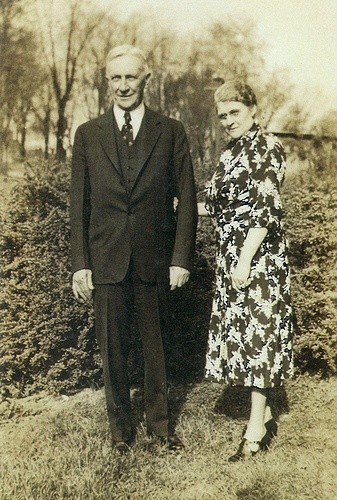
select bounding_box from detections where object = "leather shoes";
[111,440,131,457]
[144,428,187,452]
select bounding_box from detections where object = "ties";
[120,111,137,147]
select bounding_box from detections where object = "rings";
[240,282,243,285]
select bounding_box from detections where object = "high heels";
[242,419,278,440]
[226,428,271,463]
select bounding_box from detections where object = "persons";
[206,80,292,461]
[70,44,198,454]
